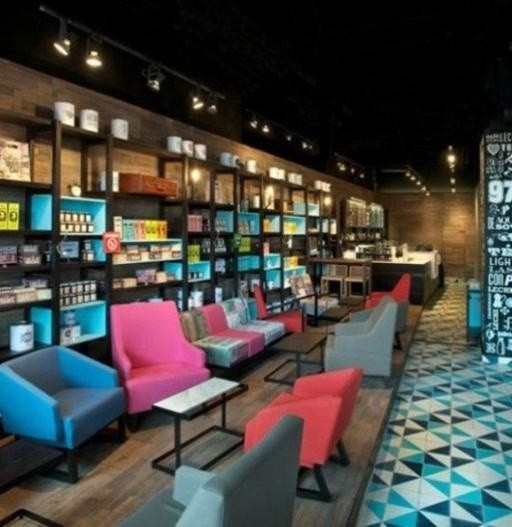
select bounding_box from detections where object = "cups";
[219,151,257,173]
[182,138,195,157]
[269,165,303,186]
[80,108,99,132]
[194,143,207,162]
[52,100,75,126]
[166,134,181,154]
[314,178,332,192]
[110,116,129,141]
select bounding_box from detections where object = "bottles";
[263,239,270,255]
[401,242,410,262]
[263,218,271,234]
[59,279,98,308]
[80,240,96,262]
[9,319,35,351]
[59,209,96,234]
[237,218,256,234]
[254,192,260,210]
[61,310,81,342]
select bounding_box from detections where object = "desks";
[309,256,373,310]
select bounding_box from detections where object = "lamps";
[51,17,228,115]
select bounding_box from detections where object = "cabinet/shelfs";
[338,198,389,257]
[0,106,340,440]
[465,284,481,344]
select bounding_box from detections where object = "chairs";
[320,264,348,299]
[343,264,372,310]
